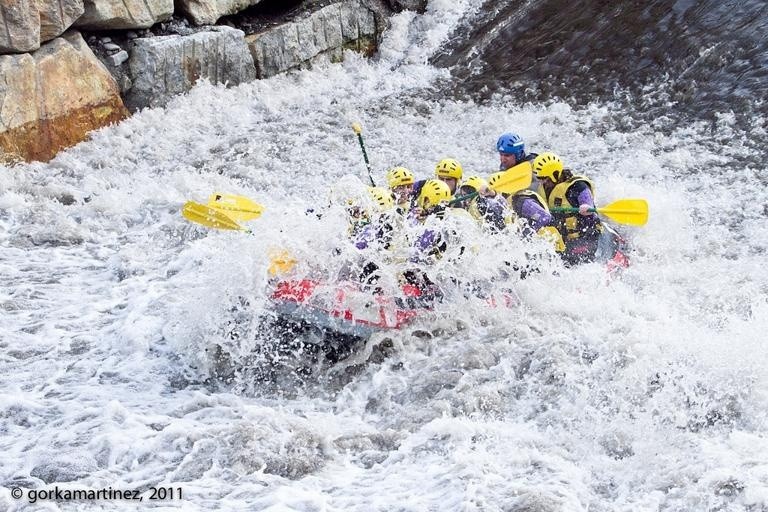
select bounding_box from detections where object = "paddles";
[548,201,648,227]
[448,160,533,207]
[183,204,247,231]
[206,192,263,224]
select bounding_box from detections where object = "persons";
[304,129,605,301]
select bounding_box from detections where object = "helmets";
[533,150,564,183]
[346,181,393,227]
[497,132,525,156]
[417,179,451,211]
[386,166,414,189]
[460,174,490,206]
[435,159,464,184]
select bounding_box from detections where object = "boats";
[272,213,629,343]
